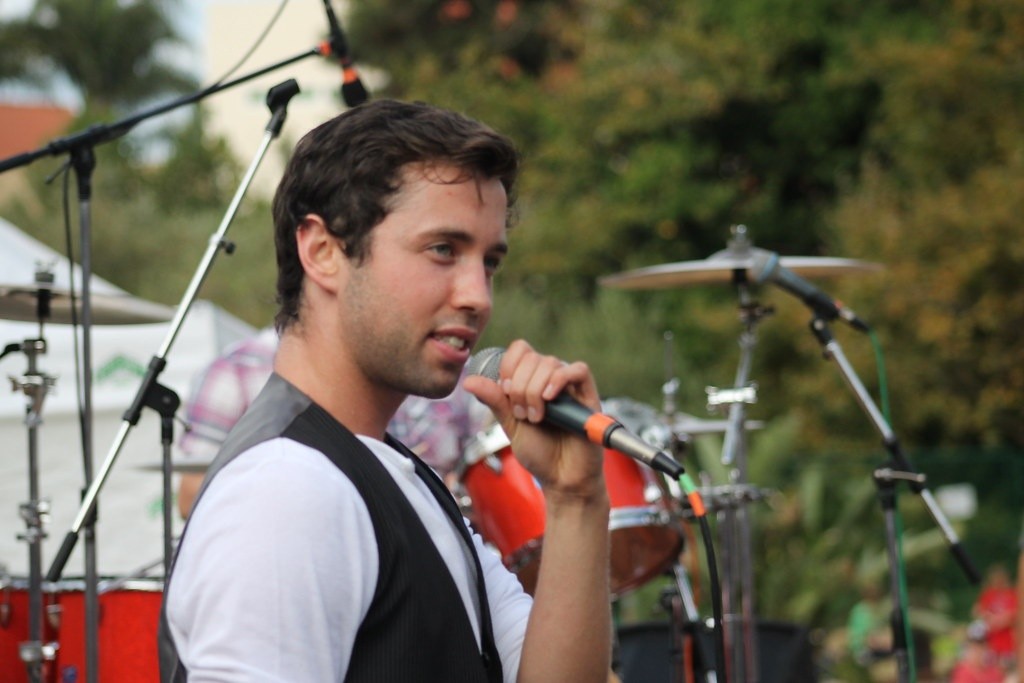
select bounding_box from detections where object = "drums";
[0,575,165,683]
[456,394,686,603]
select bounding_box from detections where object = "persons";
[849,581,893,667]
[177,324,499,523]
[966,524,1024,683]
[162,102,614,683]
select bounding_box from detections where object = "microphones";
[747,250,872,335]
[470,346,684,480]
[323,0,367,109]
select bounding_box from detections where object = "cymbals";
[0,283,175,325]
[596,244,882,288]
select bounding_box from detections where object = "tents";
[0,219,262,586]
